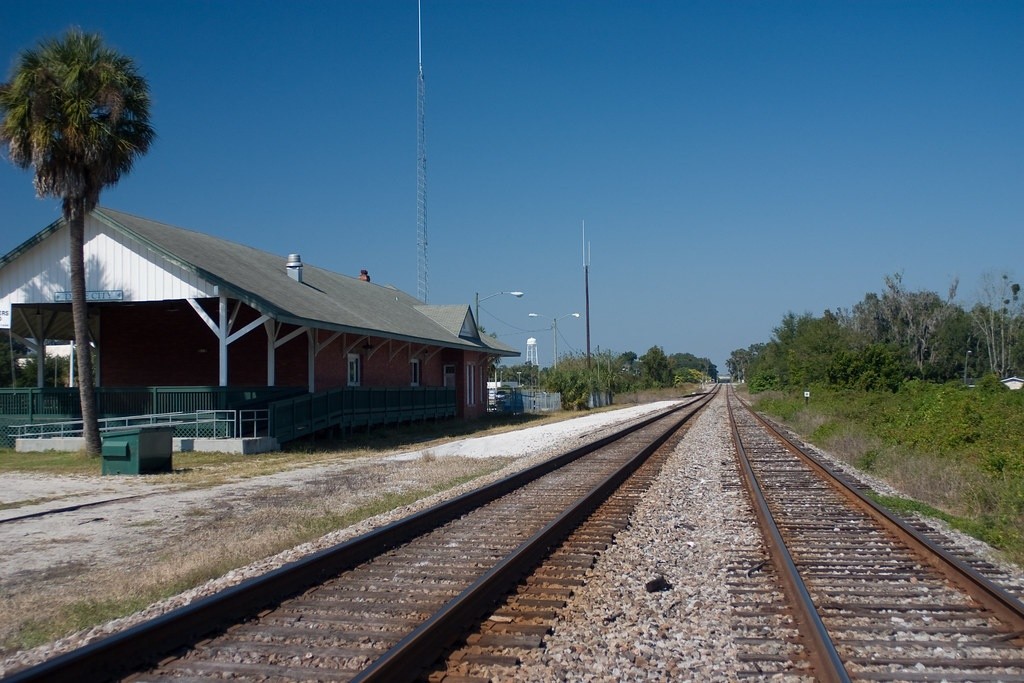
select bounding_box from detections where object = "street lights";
[964,350,972,384]
[476,290,525,330]
[529,313,580,369]
